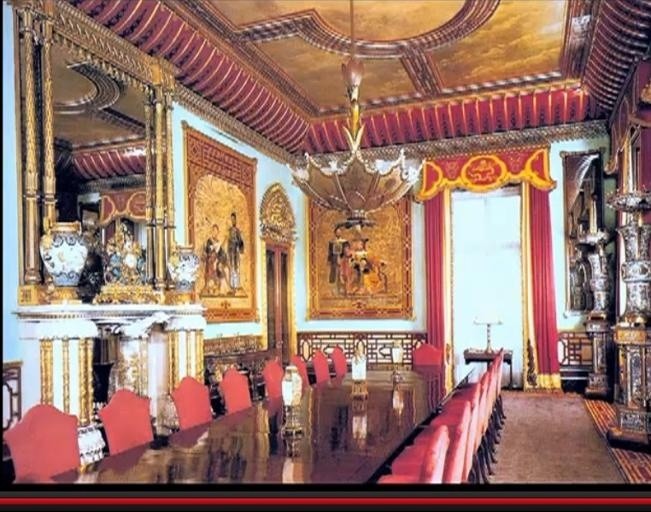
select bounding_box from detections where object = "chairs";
[97,390,156,456]
[411,342,444,366]
[378,425,451,483]
[220,368,253,415]
[262,359,284,399]
[412,382,479,481]
[4,403,82,483]
[170,376,214,431]
[314,350,331,384]
[392,400,472,482]
[292,355,309,389]
[333,346,348,379]
[431,347,507,482]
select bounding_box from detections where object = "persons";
[228,214,251,295]
[327,225,385,296]
[203,220,225,296]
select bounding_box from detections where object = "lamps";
[288,4,428,222]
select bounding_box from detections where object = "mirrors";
[6,0,182,304]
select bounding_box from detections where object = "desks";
[463,346,515,391]
[17,364,478,482]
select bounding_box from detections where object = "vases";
[166,244,201,292]
[40,221,89,287]
